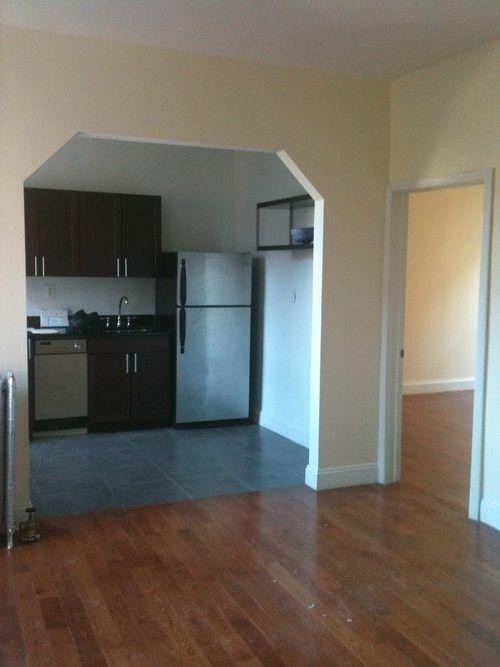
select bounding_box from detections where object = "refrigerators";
[156,250,254,427]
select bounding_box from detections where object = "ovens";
[33,341,88,421]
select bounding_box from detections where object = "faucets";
[116,296,128,325]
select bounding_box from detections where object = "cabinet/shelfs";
[87,339,176,434]
[21,187,161,278]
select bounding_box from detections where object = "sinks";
[104,327,148,333]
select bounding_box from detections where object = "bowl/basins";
[289,227,313,247]
